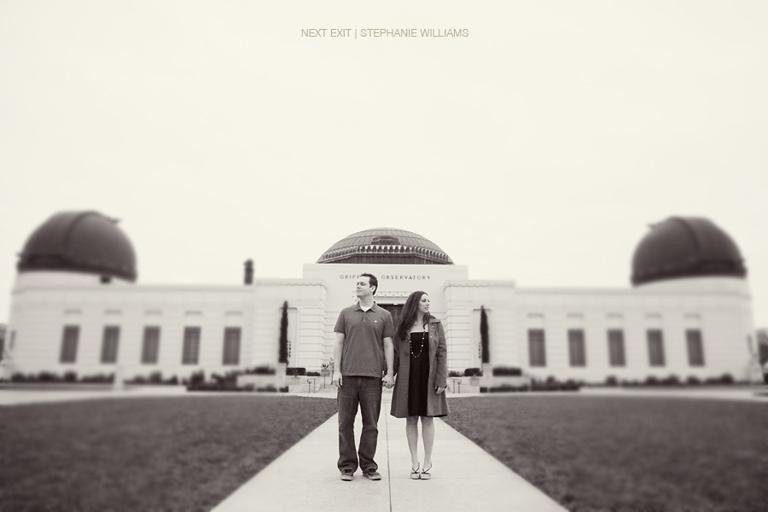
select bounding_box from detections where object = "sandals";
[411,465,420,479]
[421,464,432,479]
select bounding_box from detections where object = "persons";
[383,291,450,480]
[333,273,395,481]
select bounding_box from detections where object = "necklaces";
[408,325,425,358]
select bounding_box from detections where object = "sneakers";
[341,469,354,481]
[363,470,381,479]
